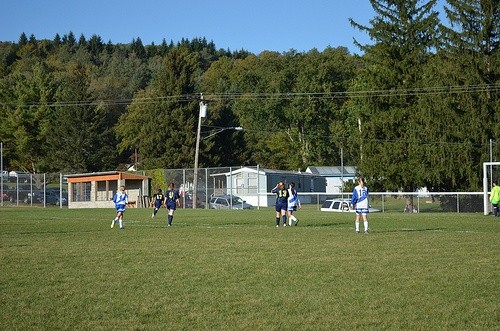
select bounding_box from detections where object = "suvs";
[320,198,381,212]
[209,194,254,210]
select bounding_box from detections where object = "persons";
[271,181,289,227]
[110,186,128,229]
[351,176,370,234]
[489,180,500,217]
[288,182,301,226]
[150,189,164,218]
[163,183,181,226]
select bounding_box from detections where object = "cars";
[175,194,205,209]
[0,170,68,206]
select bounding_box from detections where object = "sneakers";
[151,212,154,218]
[283,224,286,227]
[276,225,278,228]
[110,219,115,228]
[288,224,291,226]
[167,224,170,226]
[365,230,369,234]
[294,220,299,226]
[356,230,360,233]
[119,224,123,229]
